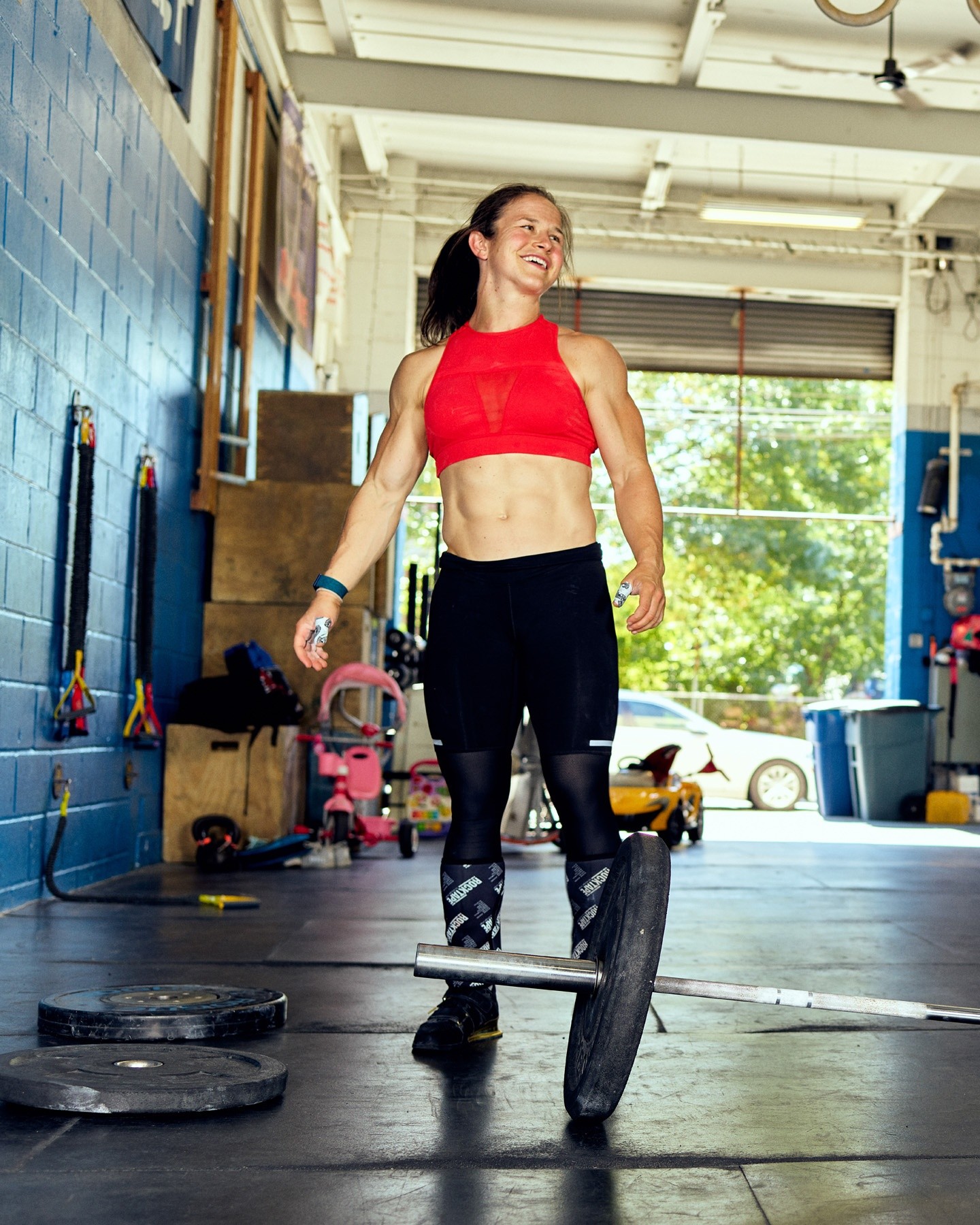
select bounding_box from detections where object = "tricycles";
[295,662,423,861]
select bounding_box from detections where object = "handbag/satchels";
[175,641,306,734]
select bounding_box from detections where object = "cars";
[612,688,819,813]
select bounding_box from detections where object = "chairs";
[340,746,382,798]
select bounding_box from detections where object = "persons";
[292,183,666,1052]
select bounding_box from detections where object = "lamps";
[343,0,867,231]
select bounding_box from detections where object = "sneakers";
[411,983,503,1054]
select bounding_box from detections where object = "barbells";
[413,831,980,1124]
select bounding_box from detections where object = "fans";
[770,12,980,121]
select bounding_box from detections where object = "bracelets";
[312,574,348,602]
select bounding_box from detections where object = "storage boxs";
[160,723,307,865]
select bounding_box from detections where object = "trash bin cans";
[841,701,943,822]
[803,699,854,817]
